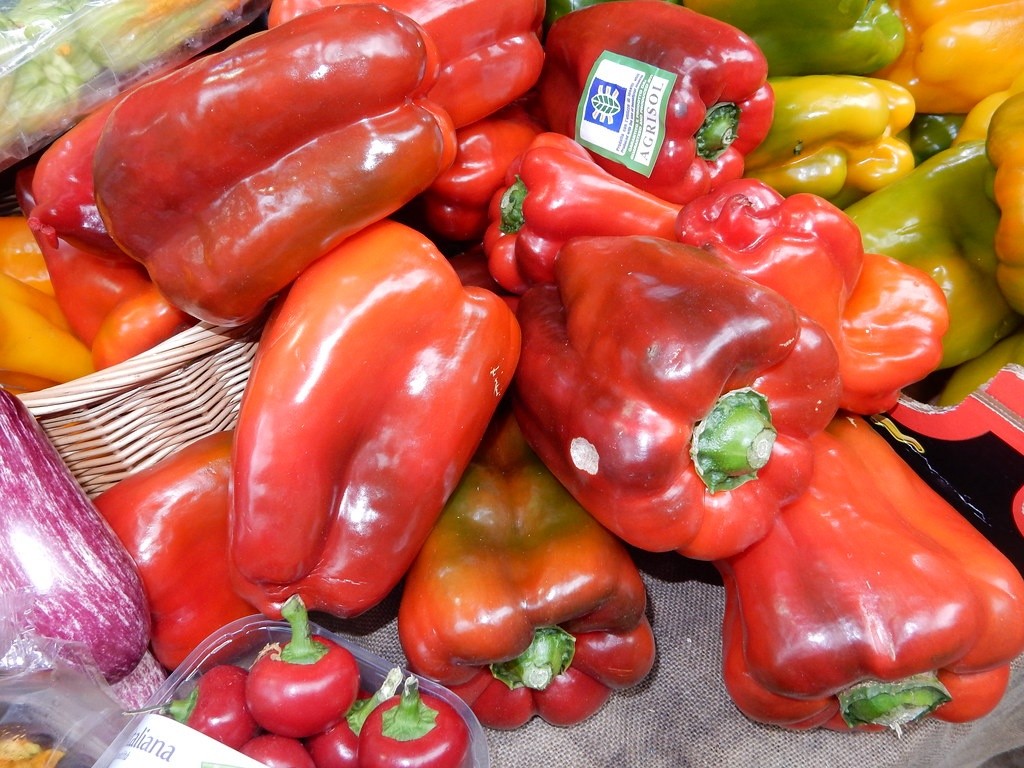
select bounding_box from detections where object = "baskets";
[16,318,270,500]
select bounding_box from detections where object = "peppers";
[0,0,1024,768]
